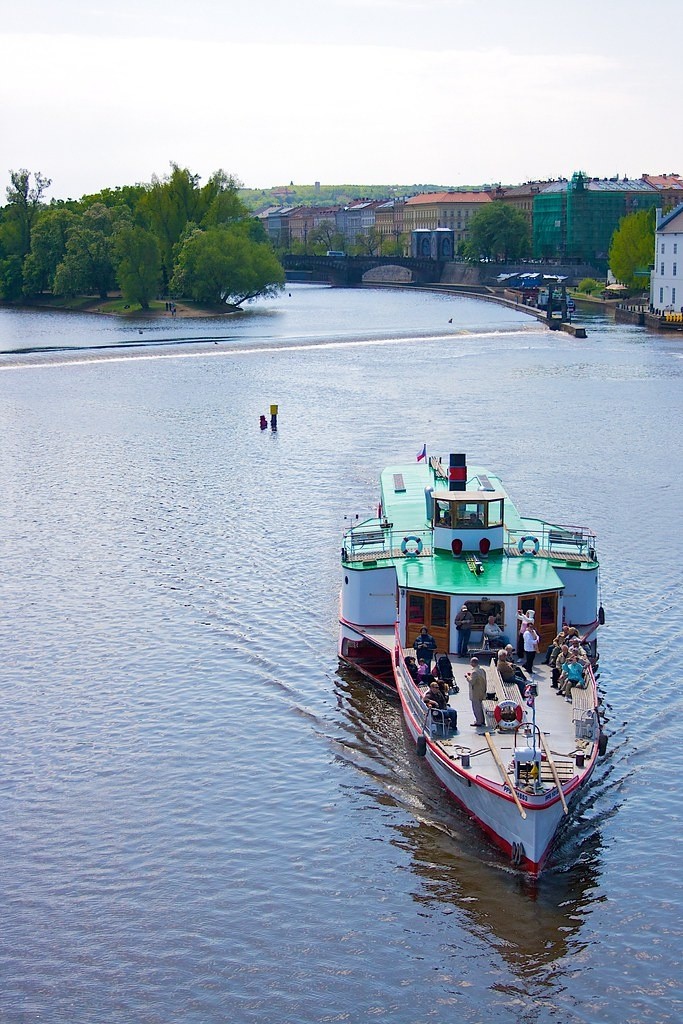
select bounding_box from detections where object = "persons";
[541,626,590,702]
[497,644,533,698]
[405,656,436,687]
[467,513,483,526]
[484,616,516,652]
[515,610,539,674]
[423,680,459,734]
[464,657,486,726]
[413,626,437,674]
[455,605,475,658]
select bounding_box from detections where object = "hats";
[461,606,467,611]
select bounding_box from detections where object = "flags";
[416,447,425,462]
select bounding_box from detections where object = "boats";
[504,287,575,313]
[338,443,609,881]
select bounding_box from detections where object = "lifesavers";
[517,535,541,557]
[494,699,524,730]
[400,535,424,557]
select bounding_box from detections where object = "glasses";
[573,642,578,645]
[432,687,440,689]
[528,626,534,629]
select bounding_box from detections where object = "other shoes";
[462,654,468,657]
[557,690,562,695]
[470,721,485,726]
[453,730,460,735]
[512,648,515,652]
[564,696,572,702]
[517,657,525,663]
[458,654,461,658]
[551,685,558,688]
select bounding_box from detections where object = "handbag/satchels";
[456,624,462,630]
